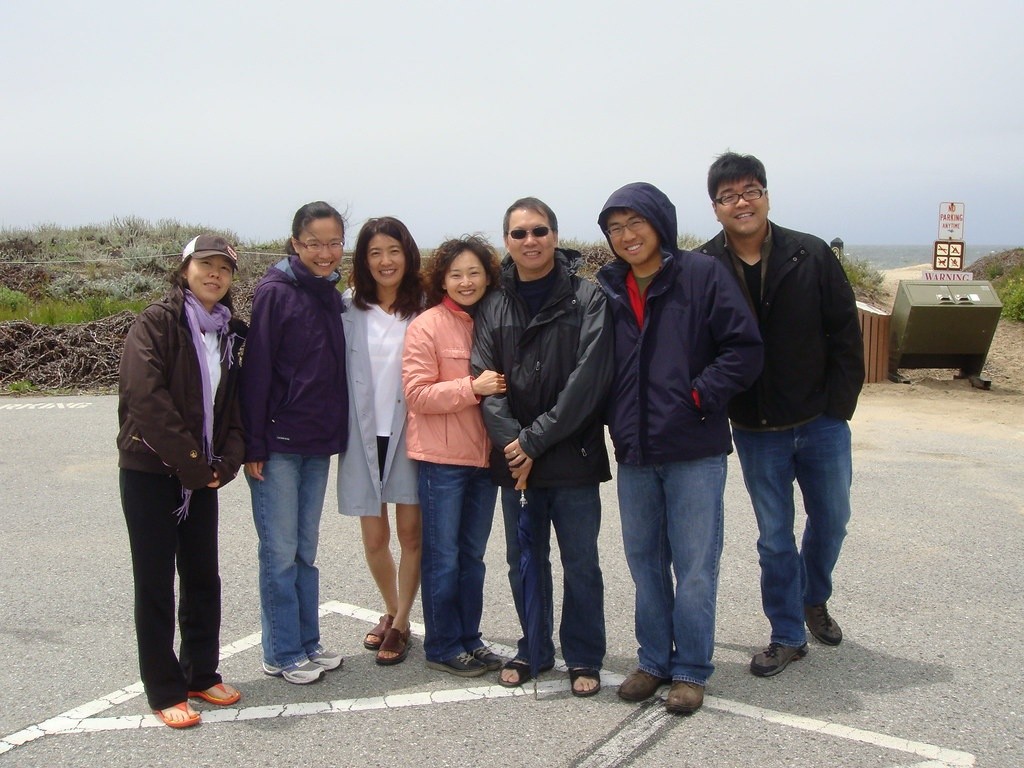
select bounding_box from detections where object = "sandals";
[376,627,412,663]
[364,614,397,648]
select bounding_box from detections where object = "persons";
[240,201,349,685]
[594,181,761,716]
[469,196,614,697]
[336,216,421,666]
[116,234,248,728]
[402,232,506,677]
[693,153,864,677]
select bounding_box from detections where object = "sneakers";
[426,651,487,677]
[750,642,808,676]
[619,668,666,699]
[466,644,502,670]
[666,680,704,712]
[263,657,325,684]
[804,601,842,645]
[307,647,343,671]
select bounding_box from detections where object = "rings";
[514,450,517,455]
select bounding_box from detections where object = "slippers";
[569,667,600,696]
[498,657,555,686]
[187,683,240,705]
[152,701,199,727]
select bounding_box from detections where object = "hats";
[180,234,238,275]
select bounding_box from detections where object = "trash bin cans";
[856,300,892,383]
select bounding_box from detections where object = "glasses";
[295,238,346,252]
[604,217,645,238]
[507,227,553,239]
[714,188,766,206]
